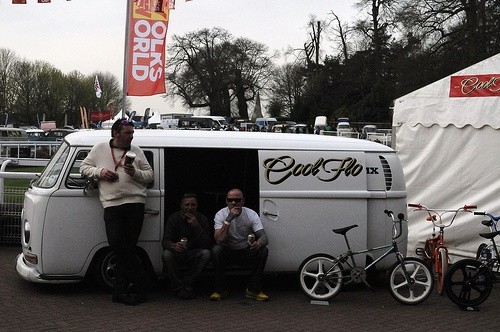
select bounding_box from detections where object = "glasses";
[227,198,243,203]
[119,120,134,127]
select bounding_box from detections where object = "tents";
[392,52,500,273]
[102,109,133,129]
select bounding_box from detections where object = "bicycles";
[442,211,500,313]
[296,208,435,306]
[407,202,478,296]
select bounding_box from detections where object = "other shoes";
[208,292,222,301]
[112,280,146,305]
[245,289,269,301]
[176,287,200,300]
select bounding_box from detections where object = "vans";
[14,127,410,296]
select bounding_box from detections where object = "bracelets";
[223,220,230,226]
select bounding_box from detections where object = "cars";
[0,112,378,157]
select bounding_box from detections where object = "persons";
[161,192,213,301]
[209,188,269,302]
[78,120,101,130]
[79,118,155,305]
[178,121,367,139]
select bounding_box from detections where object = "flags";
[143,108,150,121]
[94,75,103,98]
[127,111,136,122]
[122,0,170,97]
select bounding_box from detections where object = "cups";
[124,150,136,165]
